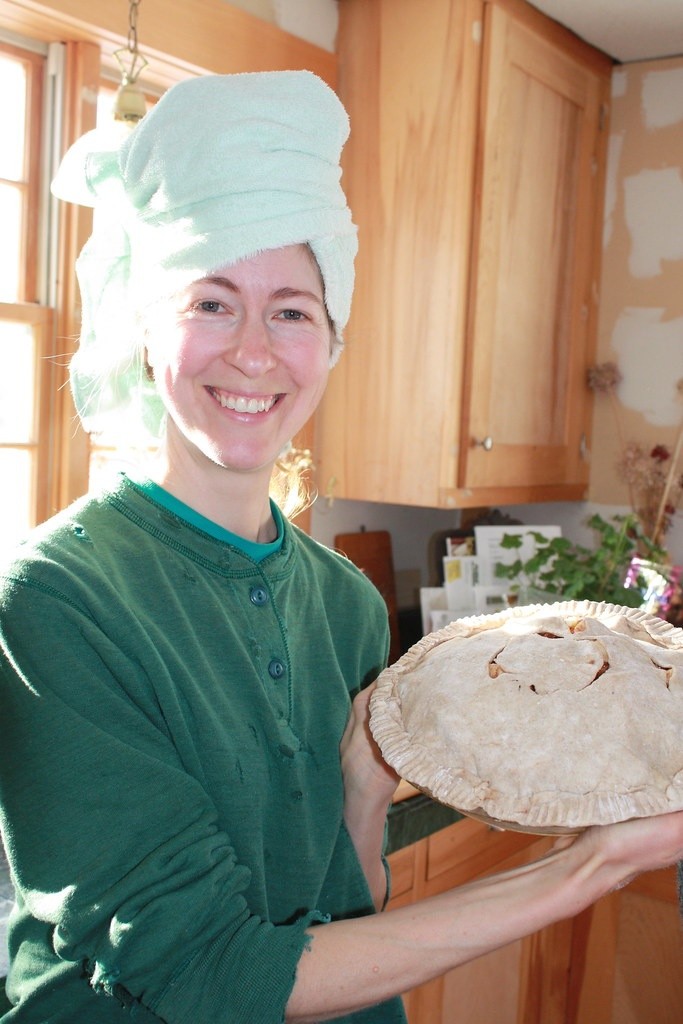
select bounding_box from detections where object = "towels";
[50,68,362,454]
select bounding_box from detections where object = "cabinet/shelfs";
[308,0,613,507]
[381,799,683,1024]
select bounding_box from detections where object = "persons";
[0,67,683,1024]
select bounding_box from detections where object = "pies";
[368,599,683,827]
